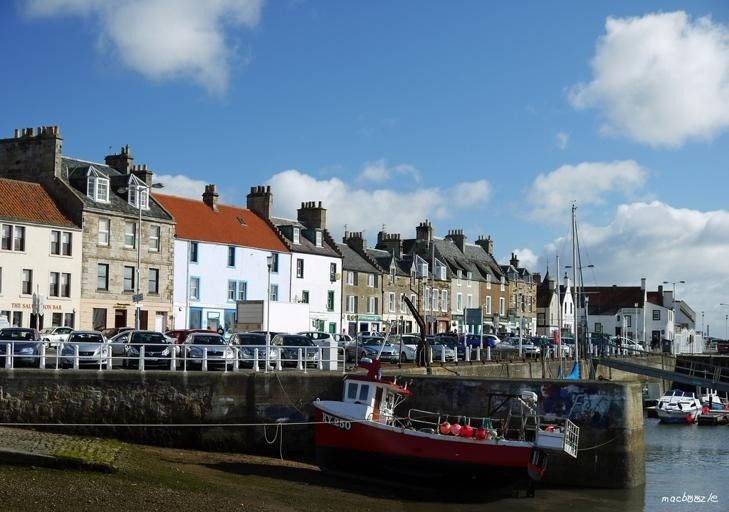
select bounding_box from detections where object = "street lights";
[416,238,453,336]
[663,281,685,311]
[513,301,532,357]
[265,256,274,334]
[117,183,164,329]
[565,265,594,310]
[634,303,638,354]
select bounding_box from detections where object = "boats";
[314,373,580,495]
[656,388,729,426]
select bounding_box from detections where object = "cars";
[495,334,644,358]
[0,327,236,368]
[229,330,501,369]
